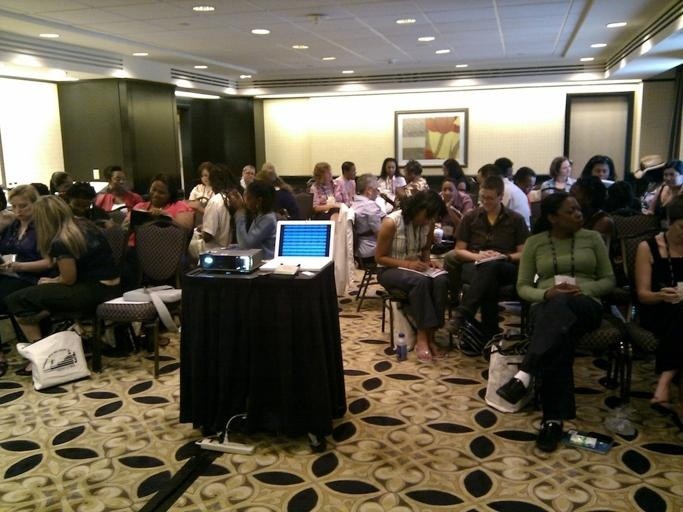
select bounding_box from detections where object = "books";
[562,427,615,455]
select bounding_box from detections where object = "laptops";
[259,220,335,272]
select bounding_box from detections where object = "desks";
[180,266,344,453]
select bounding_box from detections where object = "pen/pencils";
[417,255,425,263]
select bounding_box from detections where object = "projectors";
[197,247,262,275]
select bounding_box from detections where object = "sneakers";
[445,309,467,334]
[537,420,563,453]
[495,377,533,405]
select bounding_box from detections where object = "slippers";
[651,400,673,417]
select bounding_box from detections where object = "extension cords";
[197,438,257,456]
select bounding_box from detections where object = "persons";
[2,160,298,375]
[494,190,619,455]
[309,154,683,419]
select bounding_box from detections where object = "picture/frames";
[393,107,468,168]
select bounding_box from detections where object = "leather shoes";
[14,367,32,376]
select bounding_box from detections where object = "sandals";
[414,344,449,364]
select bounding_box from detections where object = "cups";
[1,253,16,264]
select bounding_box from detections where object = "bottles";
[570,433,610,450]
[434,226,444,245]
[395,332,408,361]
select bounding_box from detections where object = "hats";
[633,154,667,179]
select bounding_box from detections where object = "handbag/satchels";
[391,301,416,351]
[484,335,535,413]
[15,331,93,391]
